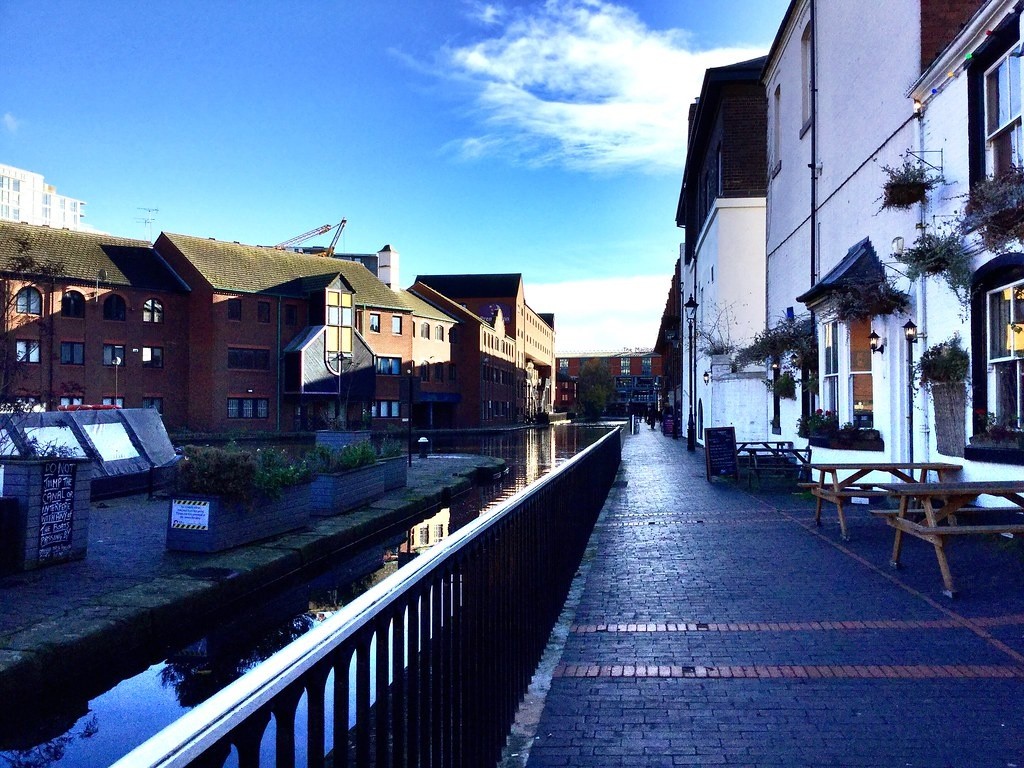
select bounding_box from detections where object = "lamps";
[901,319,927,343]
[702,372,712,385]
[789,354,801,370]
[867,330,884,354]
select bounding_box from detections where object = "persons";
[639,405,663,429]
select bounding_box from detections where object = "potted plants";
[968,409,1023,449]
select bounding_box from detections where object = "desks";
[736,441,793,455]
[810,462,962,540]
[742,448,812,464]
[876,481,1024,599]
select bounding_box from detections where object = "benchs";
[736,455,1024,591]
